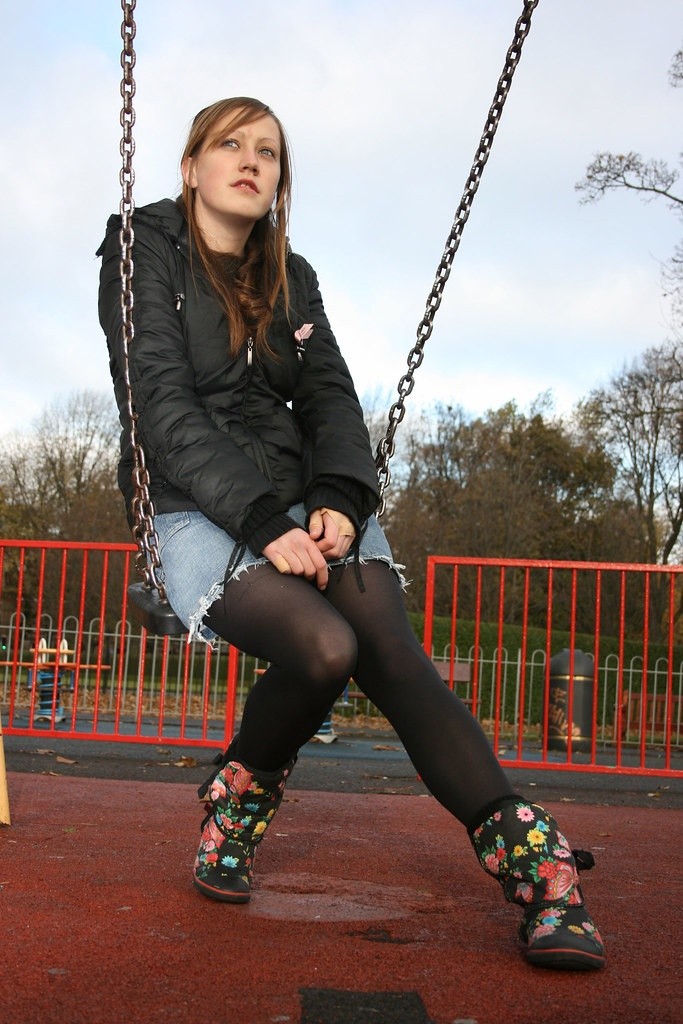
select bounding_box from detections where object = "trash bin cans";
[543,647,594,755]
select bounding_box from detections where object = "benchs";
[622,689,683,742]
[253,662,482,704]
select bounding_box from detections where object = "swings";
[114,1,545,640]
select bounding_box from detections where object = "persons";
[95,96,606,971]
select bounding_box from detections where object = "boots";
[466,796,607,971]
[191,731,297,904]
